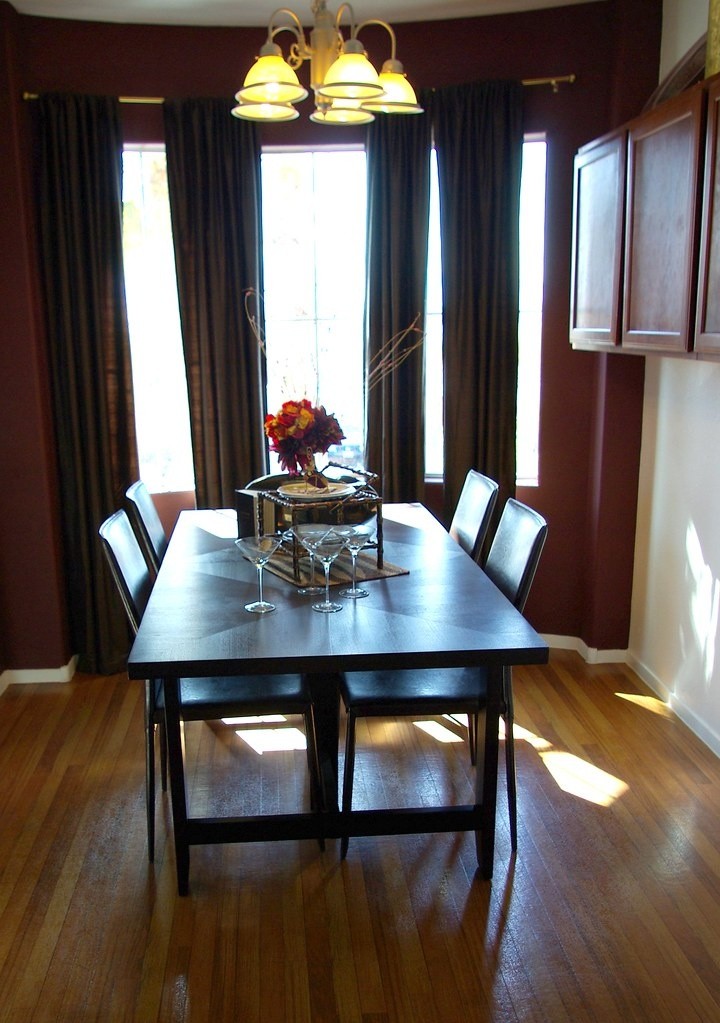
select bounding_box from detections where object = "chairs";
[125,479,169,575]
[338,498,550,865]
[447,468,500,559]
[99,508,338,861]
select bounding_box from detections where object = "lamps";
[231,0,426,128]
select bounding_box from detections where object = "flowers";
[263,397,346,481]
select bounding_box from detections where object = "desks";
[128,508,548,899]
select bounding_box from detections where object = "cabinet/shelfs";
[567,69,720,363]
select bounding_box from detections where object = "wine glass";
[303,536,350,613]
[235,536,283,614]
[332,524,376,600]
[289,523,335,596]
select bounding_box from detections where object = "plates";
[277,483,357,502]
[282,527,358,540]
